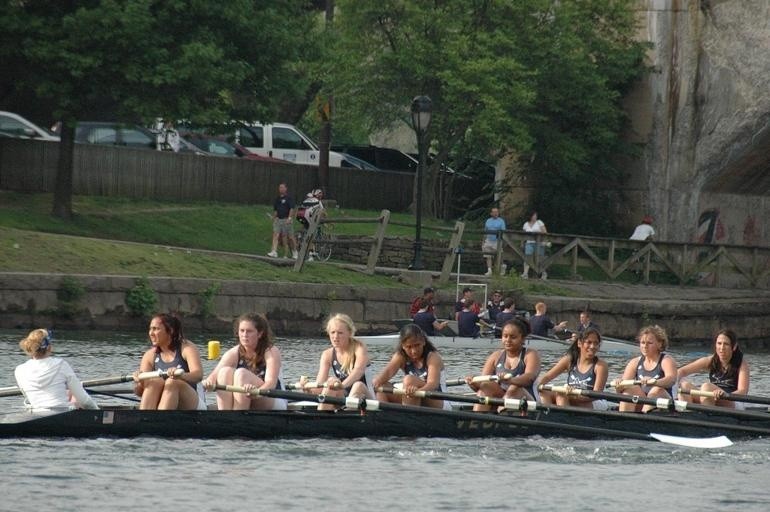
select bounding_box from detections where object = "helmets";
[311,188,323,196]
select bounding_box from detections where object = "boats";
[387,314,578,342]
[0,401,770,444]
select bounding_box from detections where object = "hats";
[640,214,656,224]
[462,287,477,294]
[493,288,505,296]
[422,287,436,295]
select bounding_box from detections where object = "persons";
[613,324,678,413]
[373,324,449,409]
[519,210,548,280]
[410,287,601,343]
[266,183,328,262]
[675,328,750,410]
[627,216,656,272]
[464,317,541,412]
[131,312,207,411]
[299,312,373,410]
[202,311,288,411]
[13,328,100,418]
[537,327,610,412]
[482,208,507,276]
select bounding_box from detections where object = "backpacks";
[296,199,320,225]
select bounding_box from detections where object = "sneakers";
[520,272,530,279]
[307,250,319,257]
[292,250,299,260]
[483,268,493,276]
[540,271,548,280]
[499,263,508,276]
[267,250,280,259]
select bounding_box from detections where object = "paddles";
[205,381,733,450]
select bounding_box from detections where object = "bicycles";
[294,221,335,264]
[625,246,661,286]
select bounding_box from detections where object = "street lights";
[407,90,434,270]
